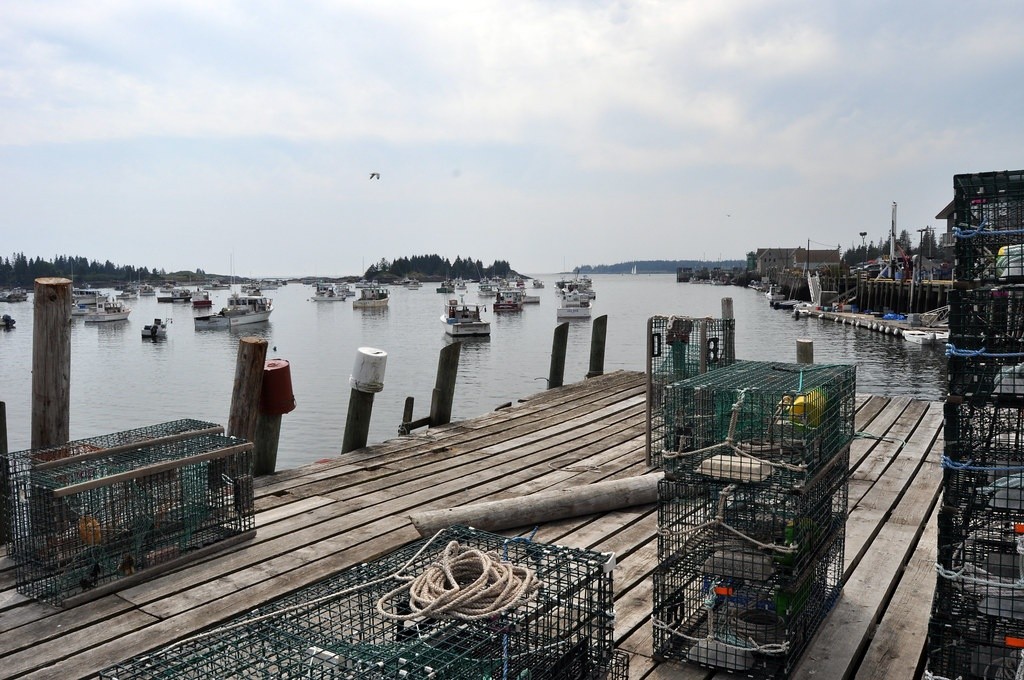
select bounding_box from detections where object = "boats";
[115,280,230,309]
[479,274,545,311]
[310,280,356,301]
[748,277,813,317]
[141,318,167,337]
[441,293,491,337]
[194,292,275,329]
[0,288,28,331]
[436,279,455,294]
[72,287,131,323]
[689,276,726,285]
[353,283,390,308]
[555,267,596,299]
[556,288,592,318]
[392,276,423,290]
[903,329,949,345]
[241,280,283,296]
[455,278,468,290]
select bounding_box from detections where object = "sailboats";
[356,255,388,288]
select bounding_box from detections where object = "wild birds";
[117,553,135,576]
[79,563,103,592]
[672,421,696,455]
